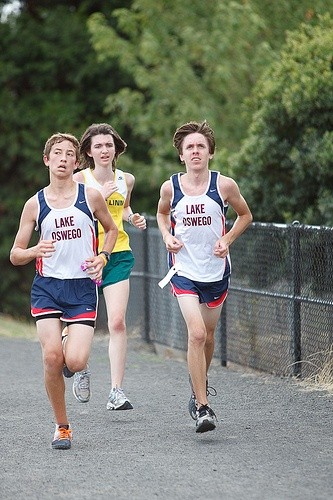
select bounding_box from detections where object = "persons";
[156,120,253,435]
[10,133,118,449]
[73,122,147,411]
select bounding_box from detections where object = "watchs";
[100,250,110,261]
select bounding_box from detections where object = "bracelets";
[128,214,133,225]
[98,254,107,266]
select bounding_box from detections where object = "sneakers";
[106,384,133,410]
[187,374,218,419]
[62,325,75,377]
[72,361,92,402]
[194,402,217,433]
[51,422,72,449]
